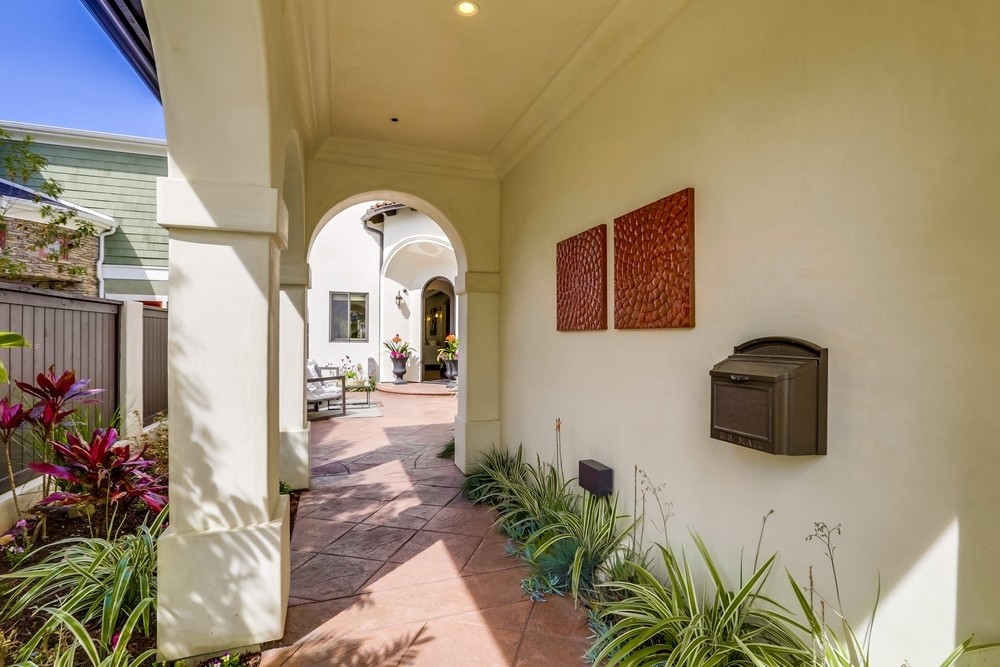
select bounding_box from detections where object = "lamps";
[395,290,402,307]
[435,306,441,318]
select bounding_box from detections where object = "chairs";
[307,359,342,410]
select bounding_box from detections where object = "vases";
[443,359,458,388]
[391,357,409,384]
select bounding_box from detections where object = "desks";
[328,381,374,410]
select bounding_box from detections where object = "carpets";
[303,399,383,418]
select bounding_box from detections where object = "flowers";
[437,335,459,368]
[383,336,417,359]
